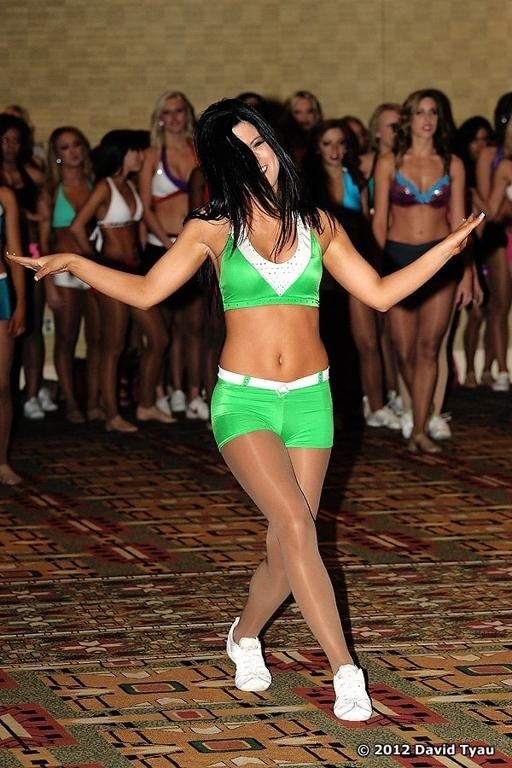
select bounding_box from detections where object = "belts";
[218,368,331,392]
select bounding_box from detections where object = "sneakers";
[226,616,273,691]
[24,386,209,432]
[0,464,22,485]
[362,390,452,453]
[465,370,509,391]
[334,664,372,722]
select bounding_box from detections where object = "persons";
[7,93,485,722]
[369,86,466,457]
[1,94,512,438]
